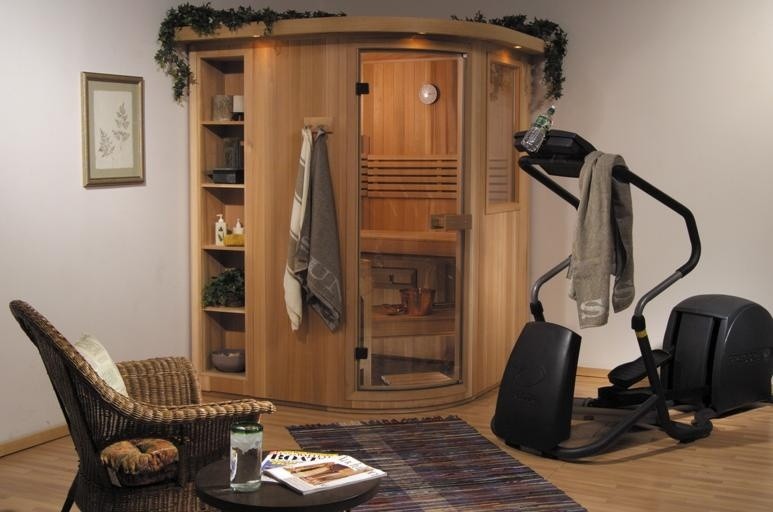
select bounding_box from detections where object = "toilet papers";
[224,226,244,245]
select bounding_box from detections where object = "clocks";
[418,82,438,107]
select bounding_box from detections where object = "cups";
[227,423,263,492]
[209,94,232,122]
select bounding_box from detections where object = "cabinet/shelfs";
[160,18,554,415]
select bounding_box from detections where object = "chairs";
[10,297,277,512]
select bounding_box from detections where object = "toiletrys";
[236,218,241,226]
[213,214,227,244]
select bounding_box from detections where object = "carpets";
[283,413,586,511]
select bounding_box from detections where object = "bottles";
[521,105,559,151]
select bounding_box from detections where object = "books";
[260,451,339,484]
[262,454,389,496]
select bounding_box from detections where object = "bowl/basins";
[210,348,245,373]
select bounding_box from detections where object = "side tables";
[193,449,382,512]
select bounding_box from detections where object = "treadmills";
[490,130,772,456]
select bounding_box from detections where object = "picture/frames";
[79,71,145,188]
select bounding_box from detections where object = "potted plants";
[202,267,245,308]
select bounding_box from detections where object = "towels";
[283,124,343,335]
[565,150,636,329]
